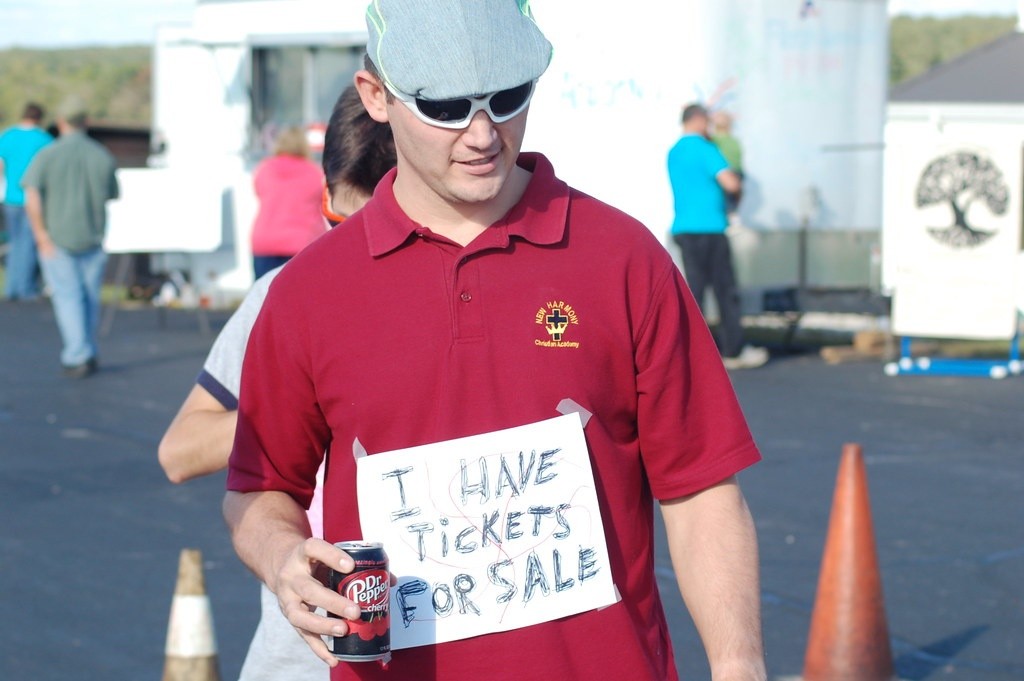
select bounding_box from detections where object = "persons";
[251,122,328,280]
[667,103,769,371]
[23,100,119,379]
[158,86,398,681]
[221,0,769,681]
[0,101,56,303]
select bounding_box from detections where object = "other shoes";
[64,361,94,379]
[721,343,769,369]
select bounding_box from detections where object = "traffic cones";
[800,442,896,681]
[164,549,217,680]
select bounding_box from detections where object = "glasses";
[376,74,538,129]
[322,185,348,229]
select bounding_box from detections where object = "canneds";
[327,540,392,662]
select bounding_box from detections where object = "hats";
[365,1,553,103]
